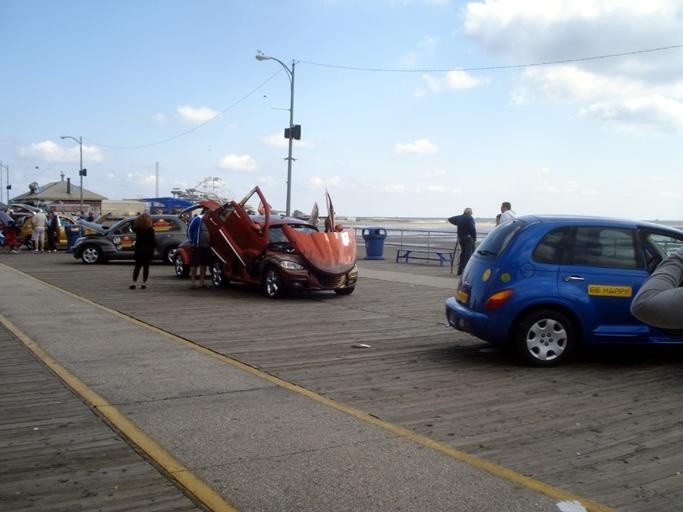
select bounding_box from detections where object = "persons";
[498,201,516,225]
[47,204,100,221]
[29,208,46,254]
[447,207,477,276]
[495,213,501,226]
[185,206,210,289]
[0,207,17,255]
[629,243,683,331]
[135,207,189,227]
[127,213,155,290]
[44,210,58,253]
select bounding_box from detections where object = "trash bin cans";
[362,227,387,260]
[64,224,84,253]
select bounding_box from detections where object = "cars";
[439,213,683,368]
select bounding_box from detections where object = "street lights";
[58,134,87,214]
[252,49,303,218]
[0,163,11,205]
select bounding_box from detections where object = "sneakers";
[129,285,146,289]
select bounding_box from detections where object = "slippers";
[189,284,207,289]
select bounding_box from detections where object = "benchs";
[395,248,455,267]
